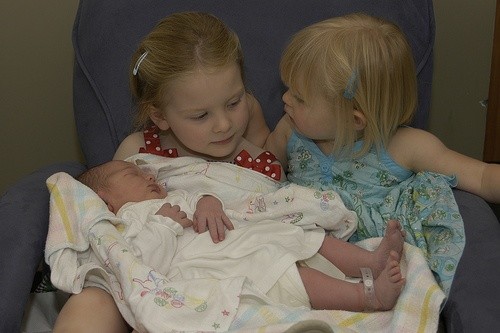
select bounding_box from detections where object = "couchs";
[0,0,500,332]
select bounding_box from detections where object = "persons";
[54,10,287,333]
[76,159,407,313]
[243,12,500,315]
